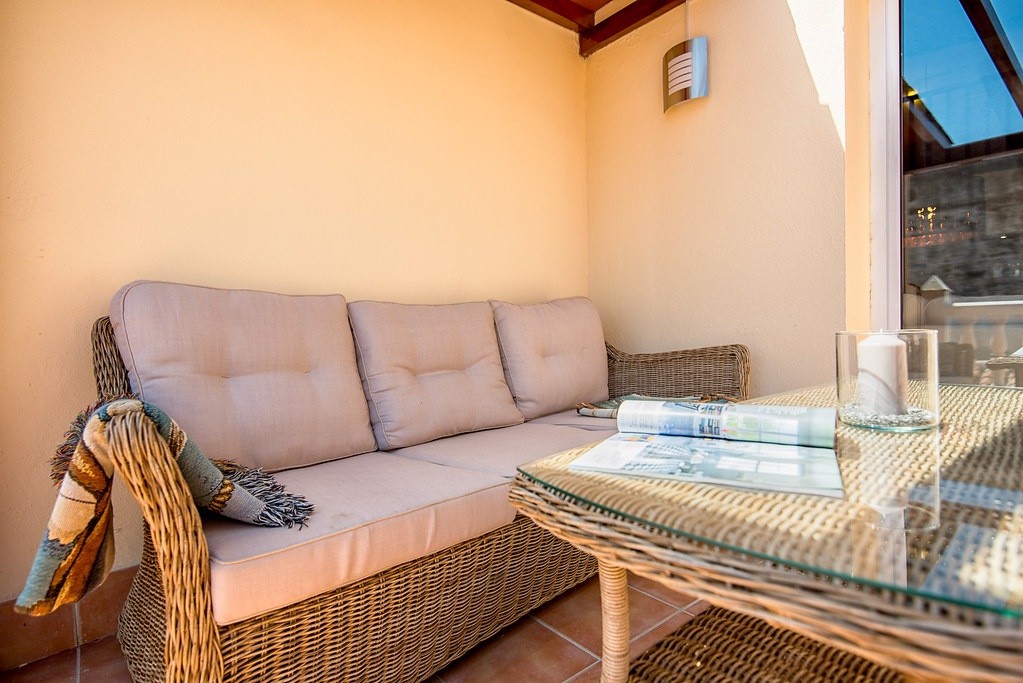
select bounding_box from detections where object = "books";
[569,399,847,498]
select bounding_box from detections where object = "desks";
[508,378,1019,683]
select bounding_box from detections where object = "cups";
[840,427,939,532]
[836,329,940,430]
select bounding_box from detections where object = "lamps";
[662,0,710,116]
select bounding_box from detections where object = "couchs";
[90,279,752,683]
